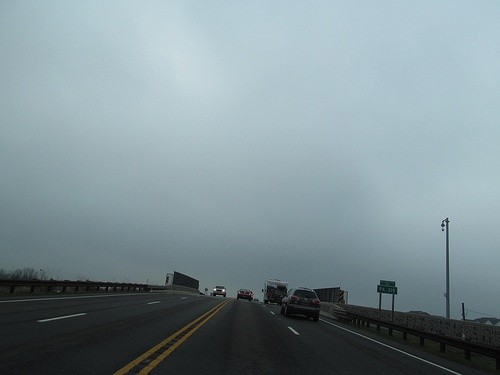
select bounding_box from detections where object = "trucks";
[262,279,287,305]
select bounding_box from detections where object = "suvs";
[213,286,226,297]
[281,286,320,321]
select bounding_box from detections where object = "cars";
[237,288,253,301]
